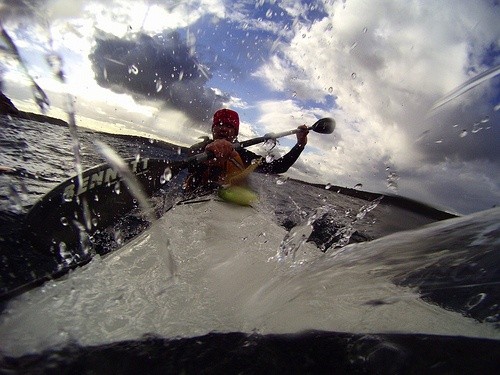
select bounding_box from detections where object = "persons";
[169,108,309,202]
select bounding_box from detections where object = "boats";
[0,154,500,375]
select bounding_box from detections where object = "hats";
[212,109,239,136]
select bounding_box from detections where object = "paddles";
[26,117,336,255]
